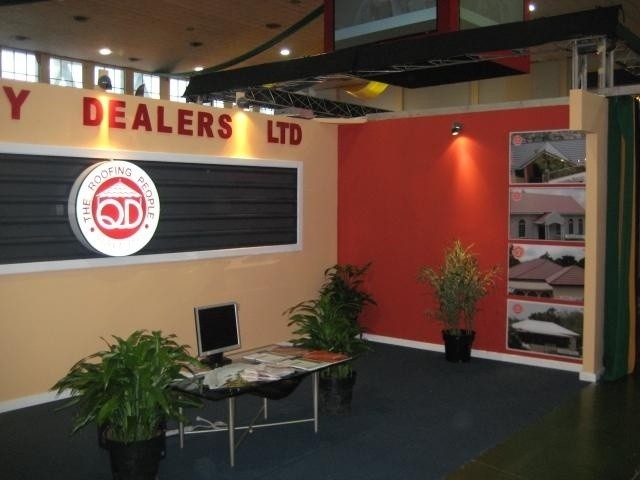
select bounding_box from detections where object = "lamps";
[98,75,112,90]
[451,120,464,136]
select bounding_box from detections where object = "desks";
[166,342,352,468]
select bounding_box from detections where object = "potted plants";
[48,329,209,479]
[281,260,378,417]
[416,239,504,363]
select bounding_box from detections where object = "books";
[247,343,350,373]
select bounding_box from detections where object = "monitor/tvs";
[191,301,241,369]
